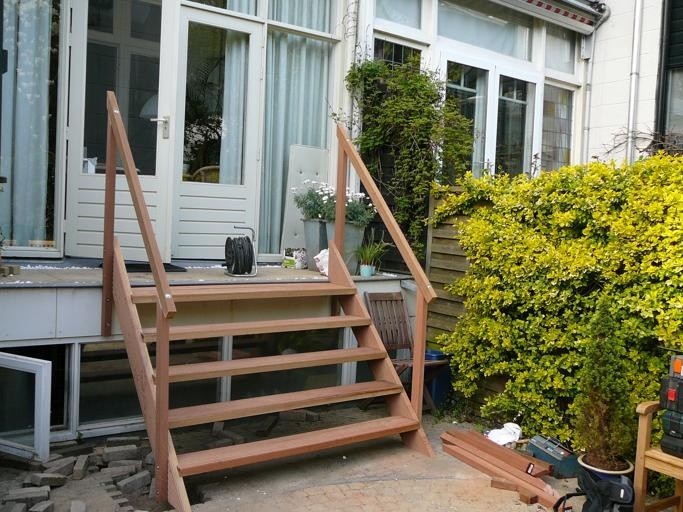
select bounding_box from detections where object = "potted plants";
[354,229,394,280]
[573,285,644,512]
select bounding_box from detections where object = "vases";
[304,218,363,277]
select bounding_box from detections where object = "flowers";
[293,177,380,221]
